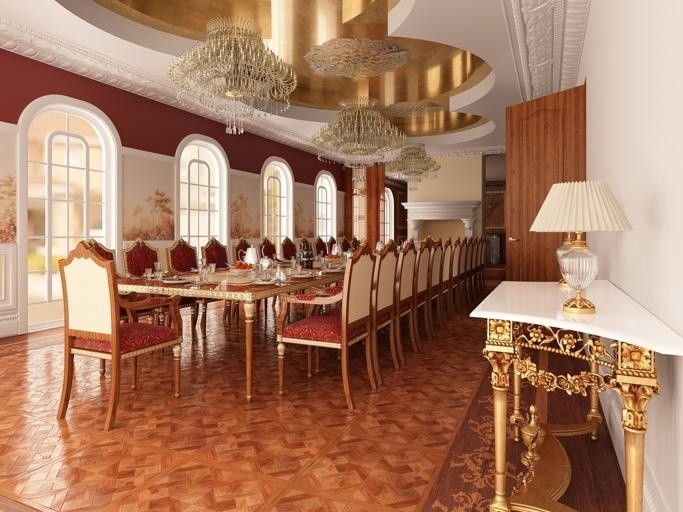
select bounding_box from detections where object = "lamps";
[309,94,411,191]
[385,143,443,191]
[167,16,300,140]
[556,232,572,292]
[526,178,634,315]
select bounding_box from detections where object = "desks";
[466,275,683,512]
[99,261,345,404]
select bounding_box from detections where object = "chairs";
[167,236,365,343]
[276,242,377,410]
[57,236,182,431]
[372,236,488,386]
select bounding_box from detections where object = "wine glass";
[142,242,355,289]
